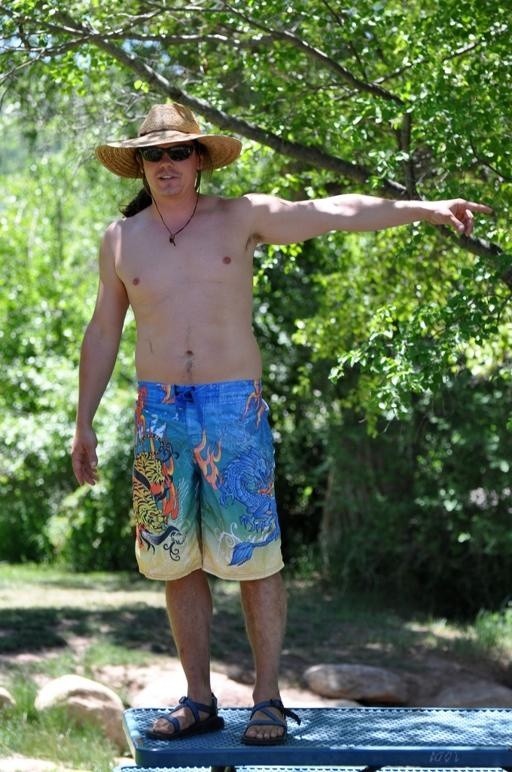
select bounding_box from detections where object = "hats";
[96,104,241,178]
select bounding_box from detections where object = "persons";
[72,102,493,745]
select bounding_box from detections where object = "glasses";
[139,144,194,161]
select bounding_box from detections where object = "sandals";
[243,699,288,744]
[146,695,224,738]
